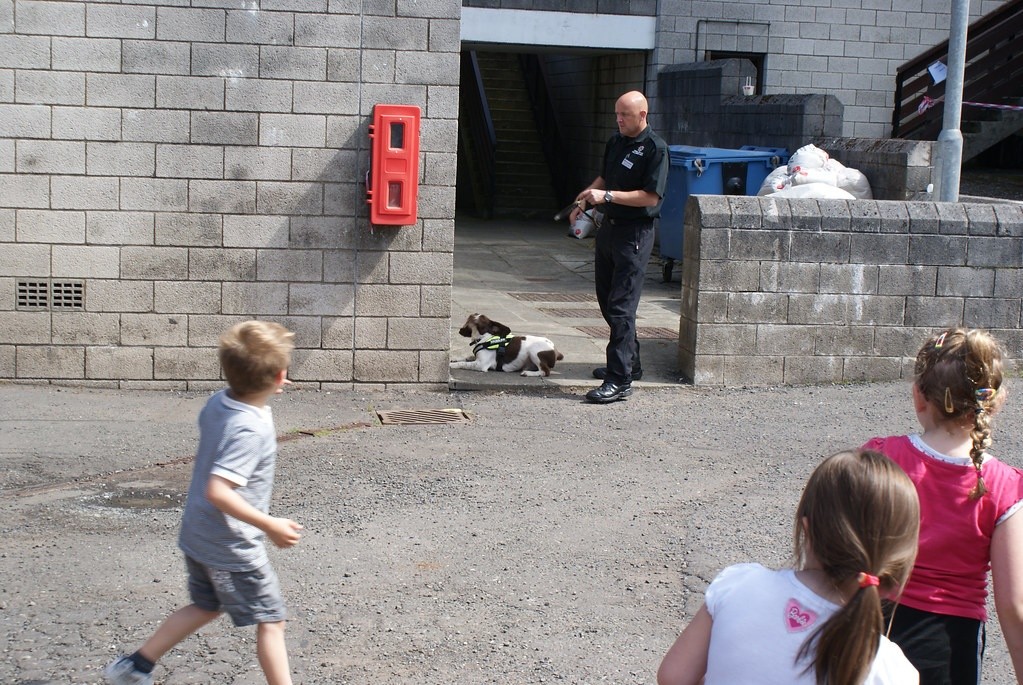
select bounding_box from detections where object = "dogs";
[451,313,564,377]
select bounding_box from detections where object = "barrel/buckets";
[742,76,755,96]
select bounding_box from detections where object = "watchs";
[603,189,613,204]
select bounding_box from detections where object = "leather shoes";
[595,368,643,380]
[586,382,633,402]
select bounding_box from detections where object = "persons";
[858,324,1023,685]
[570,89,670,405]
[655,449,926,685]
[107,319,306,685]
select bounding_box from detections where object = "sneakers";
[106,656,155,685]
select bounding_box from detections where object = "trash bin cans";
[656,145,788,282]
